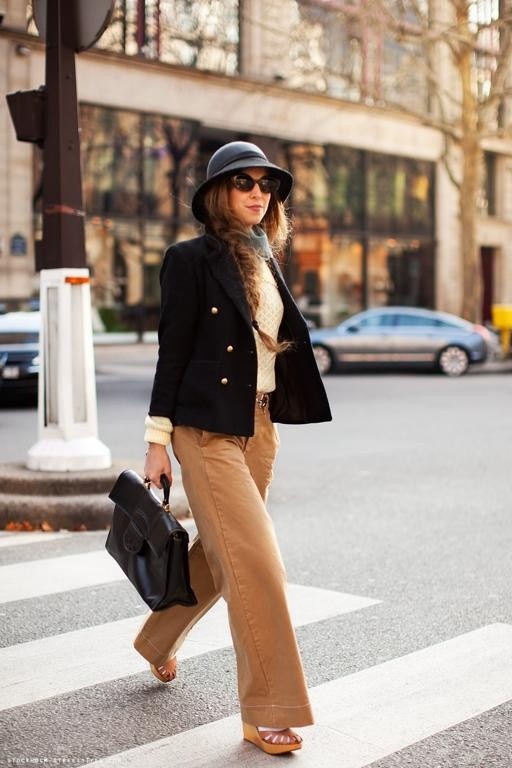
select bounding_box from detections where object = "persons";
[130,140,334,758]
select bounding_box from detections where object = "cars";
[309,304,490,376]
[0,295,39,405]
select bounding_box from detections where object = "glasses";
[230,174,280,192]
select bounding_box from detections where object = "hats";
[192,141,294,224]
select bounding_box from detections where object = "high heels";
[150,657,176,683]
[243,721,303,754]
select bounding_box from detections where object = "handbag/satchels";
[105,469,198,612]
